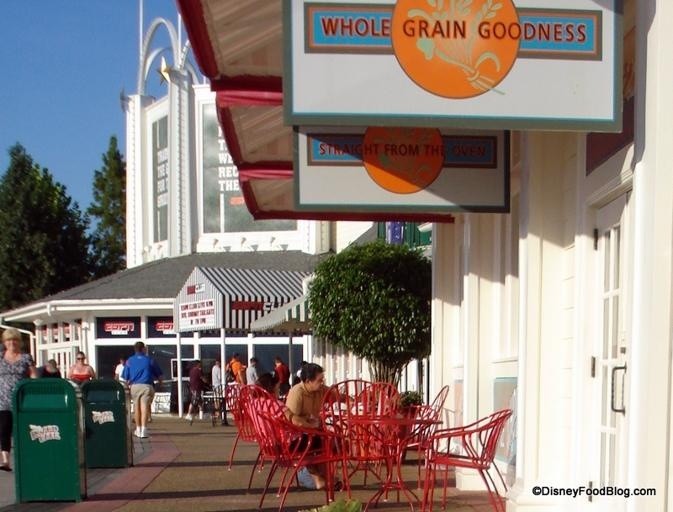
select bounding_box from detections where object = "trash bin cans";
[11,377,87,502]
[79,377,135,470]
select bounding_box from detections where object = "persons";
[297,361,308,378]
[211,359,221,418]
[121,342,164,438]
[185,360,209,421]
[38,360,61,378]
[69,352,95,381]
[226,350,246,385]
[286,364,354,490]
[246,373,339,490]
[0,328,38,472]
[273,357,291,395]
[246,358,259,385]
[114,357,126,386]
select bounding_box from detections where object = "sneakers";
[134,430,140,437]
[141,430,147,437]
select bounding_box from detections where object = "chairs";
[240,385,329,498]
[184,380,213,425]
[352,383,399,476]
[255,410,352,511]
[430,408,513,507]
[389,405,438,471]
[422,414,512,512]
[385,385,450,490]
[211,384,236,427]
[351,419,442,511]
[321,379,401,505]
[224,384,293,472]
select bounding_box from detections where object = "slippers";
[0,463,12,472]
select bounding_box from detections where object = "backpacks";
[226,361,238,382]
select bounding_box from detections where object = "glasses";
[77,358,84,360]
